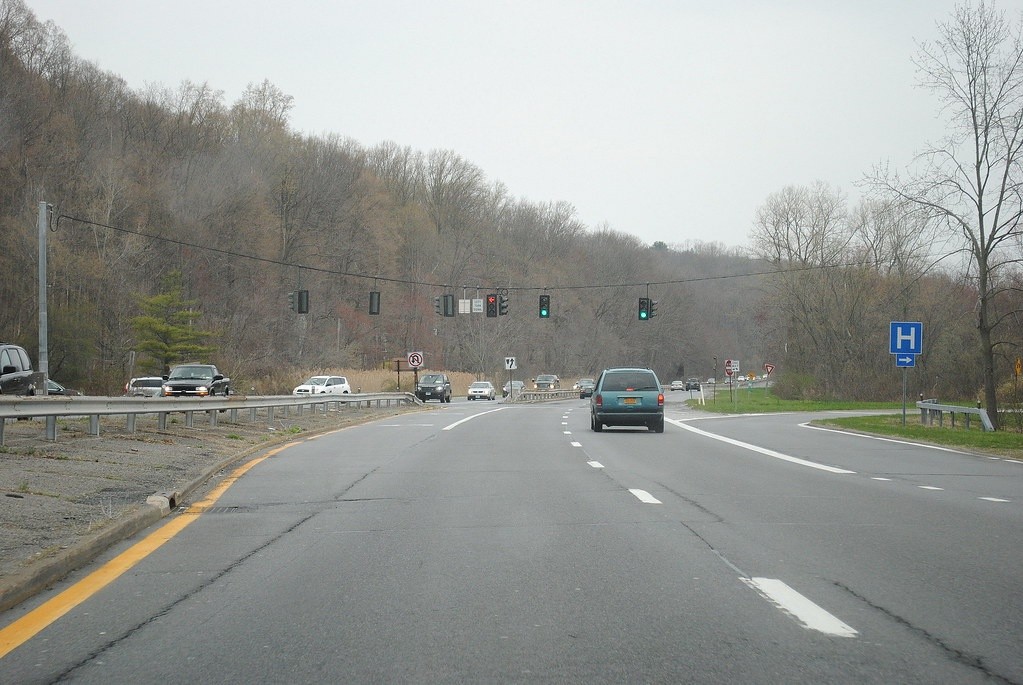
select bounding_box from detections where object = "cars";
[292,376,352,404]
[745,374,770,381]
[670,381,685,392]
[467,382,496,401]
[706,378,718,385]
[738,375,745,382]
[501,381,527,399]
[724,378,733,384]
[47,378,80,396]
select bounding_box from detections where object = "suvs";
[124,376,165,398]
[531,374,561,391]
[0,341,35,397]
[586,366,666,433]
[575,378,596,400]
[685,378,700,392]
[415,374,452,403]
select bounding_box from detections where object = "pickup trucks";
[161,364,232,414]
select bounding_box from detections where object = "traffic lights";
[487,294,498,318]
[638,297,650,321]
[499,295,509,316]
[287,290,309,315]
[539,294,552,319]
[433,294,455,318]
[725,360,736,377]
[649,298,659,319]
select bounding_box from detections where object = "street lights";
[712,356,718,404]
